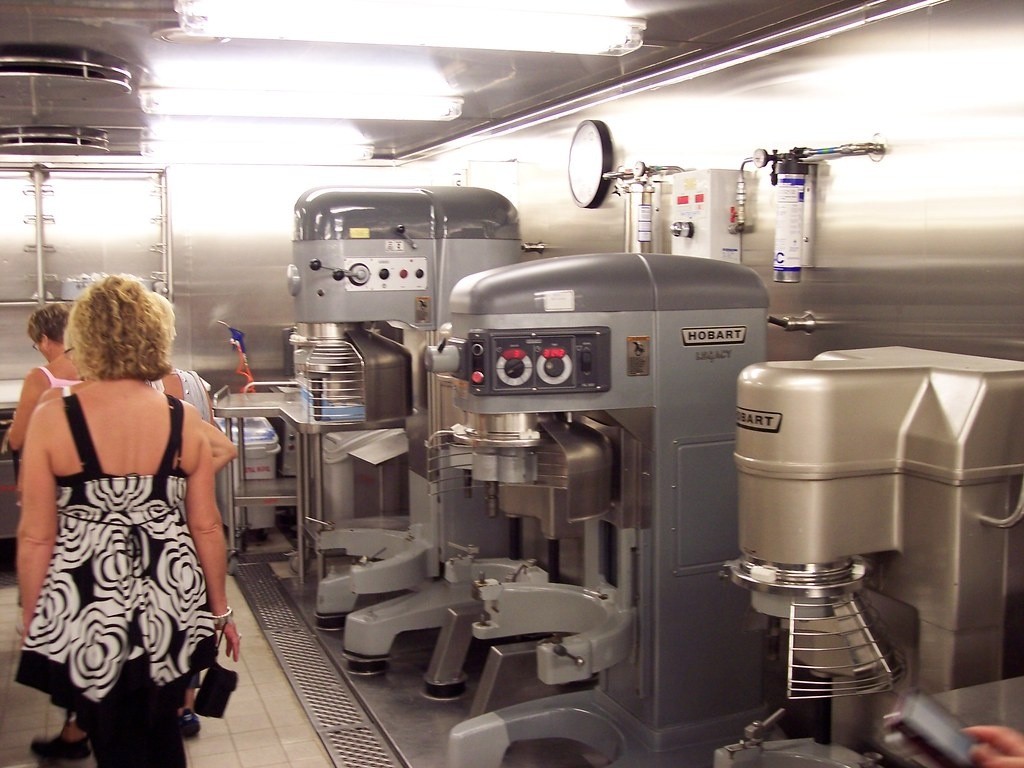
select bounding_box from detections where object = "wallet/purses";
[193,617,238,718]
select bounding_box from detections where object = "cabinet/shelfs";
[0,166,173,541]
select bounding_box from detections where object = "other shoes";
[178,709,200,738]
[30,730,91,761]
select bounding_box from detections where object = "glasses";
[33,336,41,352]
[64,346,75,360]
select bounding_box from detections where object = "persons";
[10,305,85,636]
[15,276,239,768]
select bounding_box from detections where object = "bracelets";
[213,606,233,624]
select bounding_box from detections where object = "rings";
[239,634,241,639]
